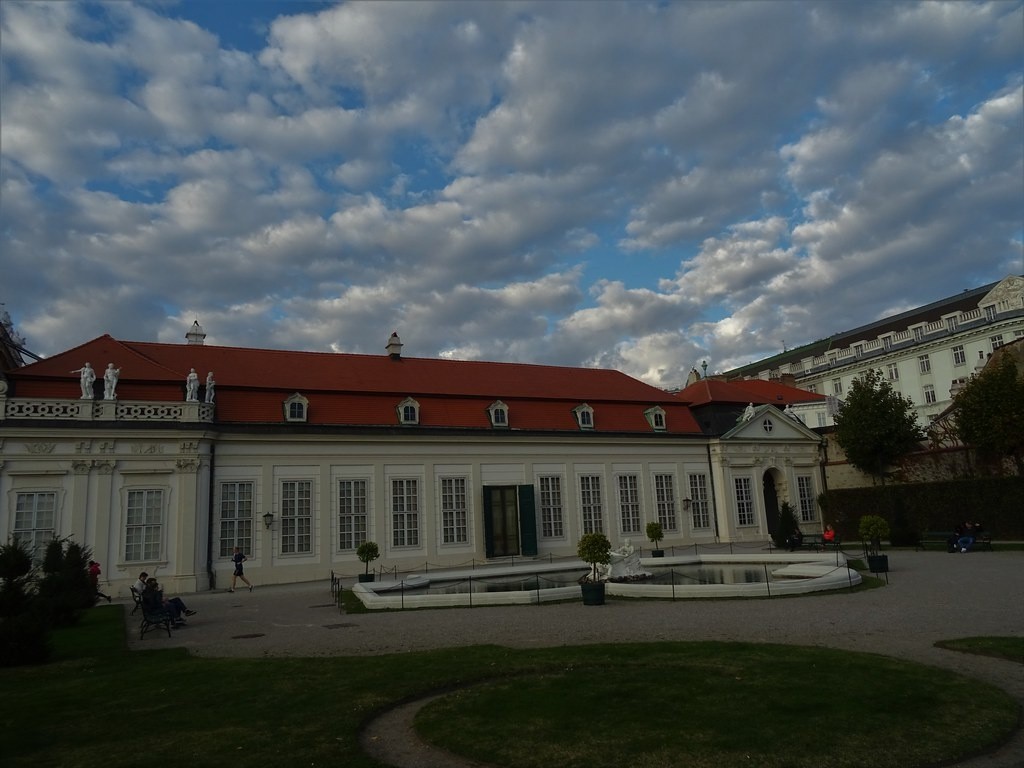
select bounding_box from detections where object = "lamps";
[263,512,273,530]
[683,497,692,511]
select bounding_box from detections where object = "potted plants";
[859,516,891,573]
[356,541,380,583]
[576,532,612,606]
[646,522,664,558]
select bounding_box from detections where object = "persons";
[186,368,200,400]
[228,547,254,593]
[743,402,753,421]
[134,572,197,622]
[103,362,120,399]
[69,362,96,399]
[954,522,979,552]
[205,372,216,403]
[88,560,112,604]
[784,404,799,424]
[613,539,636,557]
[2,311,24,346]
[788,528,803,552]
[819,524,835,552]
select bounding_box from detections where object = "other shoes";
[819,549,824,552]
[960,547,967,553]
[226,589,234,592]
[184,609,197,616]
[820,544,825,548]
[107,596,111,603]
[247,584,254,592]
[174,618,186,623]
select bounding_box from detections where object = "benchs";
[138,592,171,640]
[783,534,843,551]
[916,530,993,552]
[129,585,143,616]
